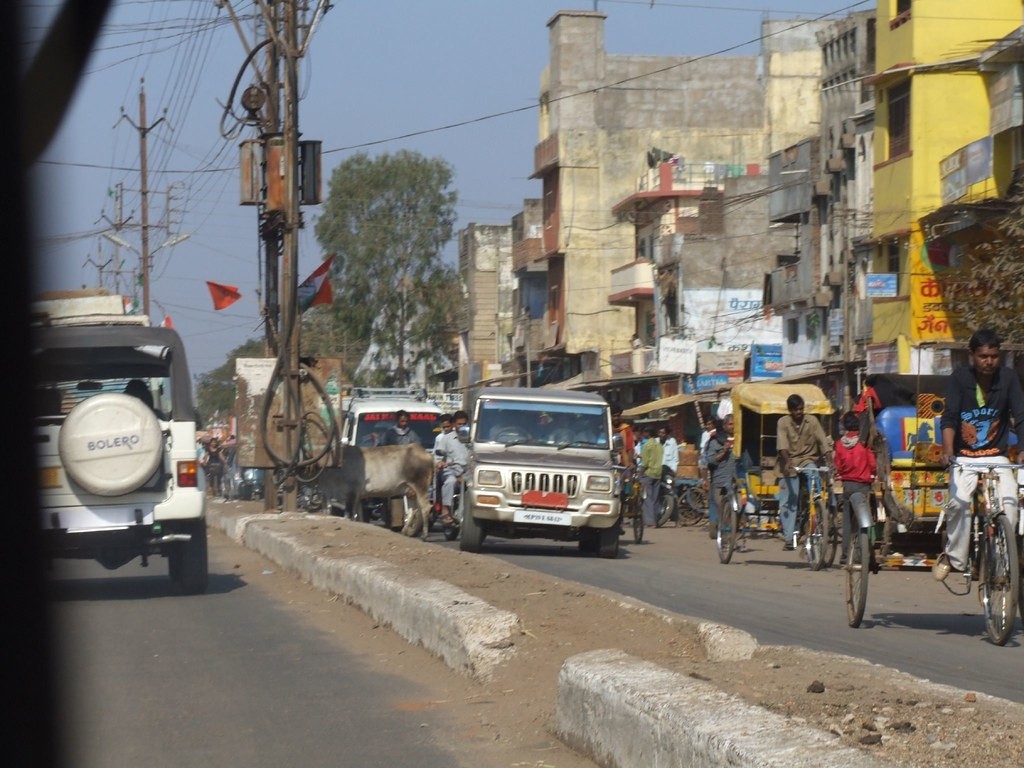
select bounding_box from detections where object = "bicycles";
[620,456,707,546]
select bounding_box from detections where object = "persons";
[932,329,1024,592]
[834,411,881,570]
[700,414,752,551]
[374,409,470,529]
[197,435,237,499]
[598,401,680,528]
[776,394,837,550]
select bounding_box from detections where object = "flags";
[159,316,174,330]
[122,297,134,316]
[296,253,336,314]
[207,281,242,310]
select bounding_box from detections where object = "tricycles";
[840,339,1023,647]
[715,382,832,571]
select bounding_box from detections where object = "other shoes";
[439,513,454,523]
[710,524,718,540]
[783,541,793,551]
[933,553,951,582]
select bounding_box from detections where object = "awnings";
[621,394,720,420]
[446,367,550,392]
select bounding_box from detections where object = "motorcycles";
[434,447,468,541]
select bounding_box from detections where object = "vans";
[339,384,444,457]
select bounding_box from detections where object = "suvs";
[32,291,209,596]
[457,386,623,558]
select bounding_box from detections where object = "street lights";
[97,233,194,319]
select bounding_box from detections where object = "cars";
[220,447,265,502]
